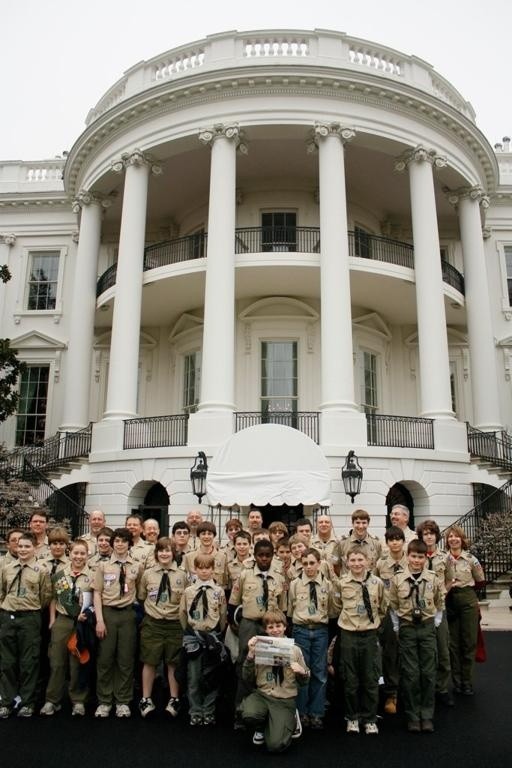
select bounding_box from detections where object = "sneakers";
[204,715,215,726]
[72,703,85,716]
[232,718,247,735]
[0,706,13,719]
[165,697,179,718]
[40,701,61,716]
[344,717,360,733]
[310,717,323,729]
[291,708,302,738]
[17,706,34,717]
[364,722,378,735]
[384,696,397,714]
[94,704,112,718]
[300,714,310,726]
[139,697,156,718]
[190,715,202,727]
[422,719,434,732]
[252,730,265,745]
[116,703,131,718]
[408,720,421,732]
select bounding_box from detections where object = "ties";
[47,559,61,578]
[403,578,422,607]
[155,569,171,605]
[189,585,210,619]
[258,574,272,612]
[174,553,183,568]
[114,560,128,599]
[298,571,324,609]
[8,565,28,596]
[353,572,374,623]
[272,666,284,686]
[393,564,399,572]
[69,573,82,602]
[426,557,434,571]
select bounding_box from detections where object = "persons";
[253,528,271,545]
[247,508,264,535]
[331,509,384,577]
[0,532,52,719]
[228,539,287,732]
[236,607,311,751]
[138,536,194,717]
[87,527,145,718]
[310,515,338,562]
[267,520,287,551]
[142,517,161,546]
[179,553,227,727]
[185,509,203,536]
[124,536,125,538]
[382,504,419,554]
[39,538,96,716]
[0,529,25,570]
[222,517,244,552]
[287,547,332,728]
[289,518,313,541]
[76,510,107,559]
[125,513,158,569]
[39,526,73,577]
[332,545,388,735]
[288,532,310,583]
[388,538,448,733]
[416,519,454,694]
[184,521,229,589]
[229,530,255,588]
[170,521,194,568]
[447,524,485,698]
[28,509,49,557]
[276,537,291,614]
[371,526,409,588]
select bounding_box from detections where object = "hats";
[228,604,243,625]
[66,630,90,664]
